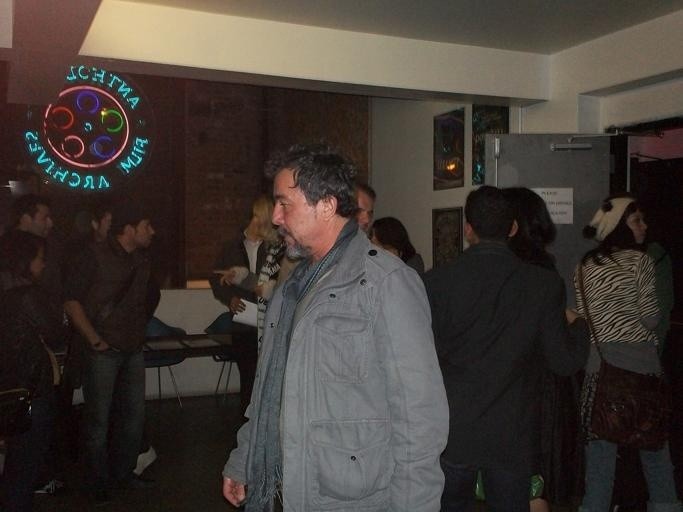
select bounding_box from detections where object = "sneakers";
[35,479,71,496]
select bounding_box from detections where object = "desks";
[143,333,233,353]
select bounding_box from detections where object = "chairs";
[207,308,249,403]
[136,316,185,413]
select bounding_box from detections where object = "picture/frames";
[430,205,464,266]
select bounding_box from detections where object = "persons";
[574,198,682,511]
[0,192,161,512]
[221,139,450,511]
[213,192,303,360]
[207,198,277,423]
[371,215,425,281]
[414,183,589,512]
[354,176,377,238]
[490,182,585,509]
[601,192,677,361]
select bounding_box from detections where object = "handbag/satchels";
[590,360,670,453]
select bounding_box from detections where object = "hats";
[582,198,635,241]
